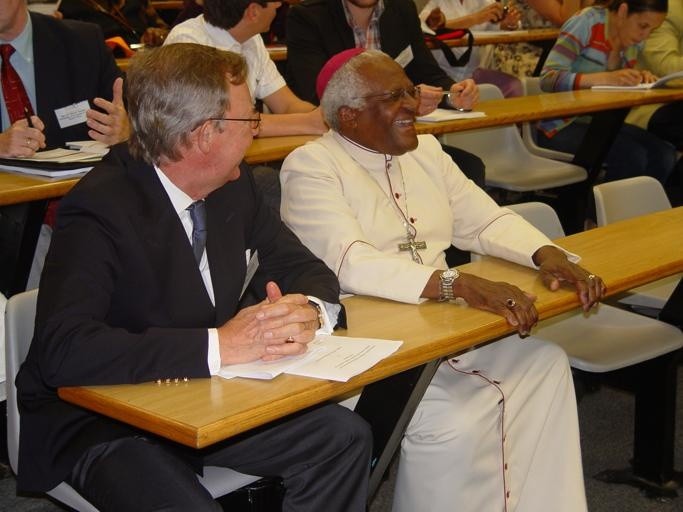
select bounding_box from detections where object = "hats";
[316,48,368,100]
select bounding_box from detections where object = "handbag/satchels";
[421,26,475,67]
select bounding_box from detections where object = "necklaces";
[334,137,427,265]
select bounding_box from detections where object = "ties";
[185,199,211,265]
[1,43,35,126]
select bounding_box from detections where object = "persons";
[14,42,375,512]
[278,47,609,512]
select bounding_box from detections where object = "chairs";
[0,72,683,512]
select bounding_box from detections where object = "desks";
[115,26,565,73]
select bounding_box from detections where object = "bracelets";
[307,297,322,331]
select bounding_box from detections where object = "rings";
[505,297,516,309]
[588,273,596,280]
[285,334,294,345]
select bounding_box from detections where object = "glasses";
[361,86,421,101]
[190,112,263,133]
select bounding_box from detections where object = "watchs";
[439,267,461,300]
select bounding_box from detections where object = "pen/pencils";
[442,90,462,94]
[24,107,33,128]
[622,55,633,69]
